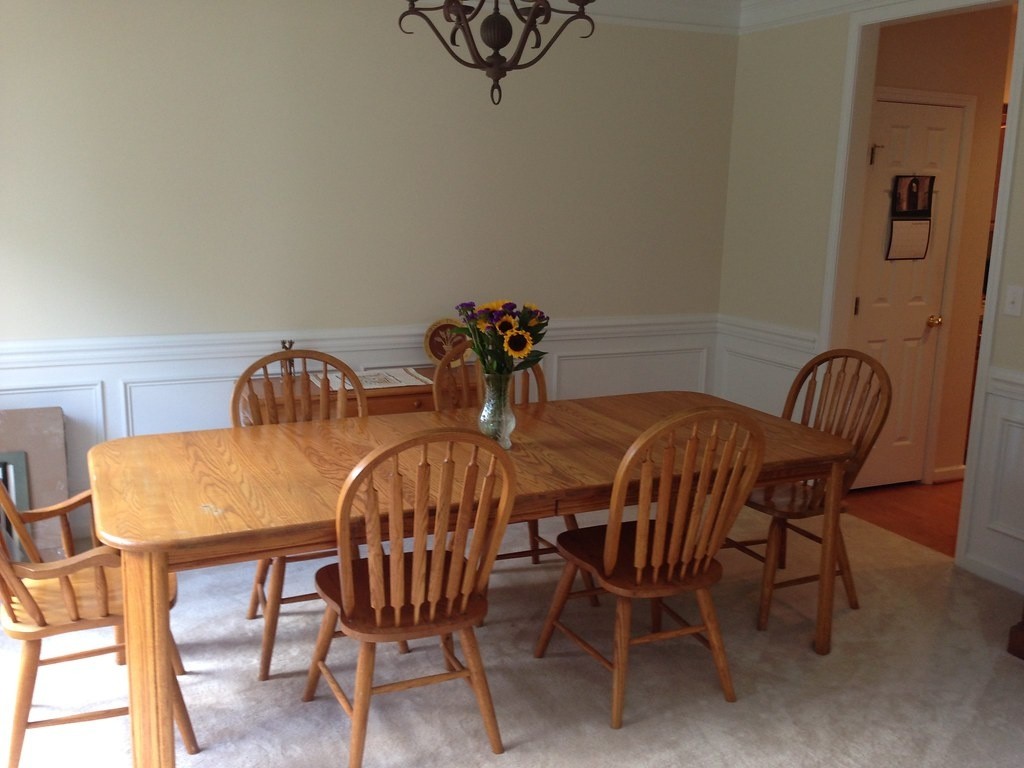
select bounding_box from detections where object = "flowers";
[453,295,552,380]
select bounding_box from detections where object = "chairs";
[698,347,892,631]
[0,476,201,768]
[228,349,412,681]
[297,427,519,765]
[534,405,766,729]
[432,337,602,627]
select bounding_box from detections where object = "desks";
[232,365,478,427]
[85,391,856,768]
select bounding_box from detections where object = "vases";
[477,372,517,452]
